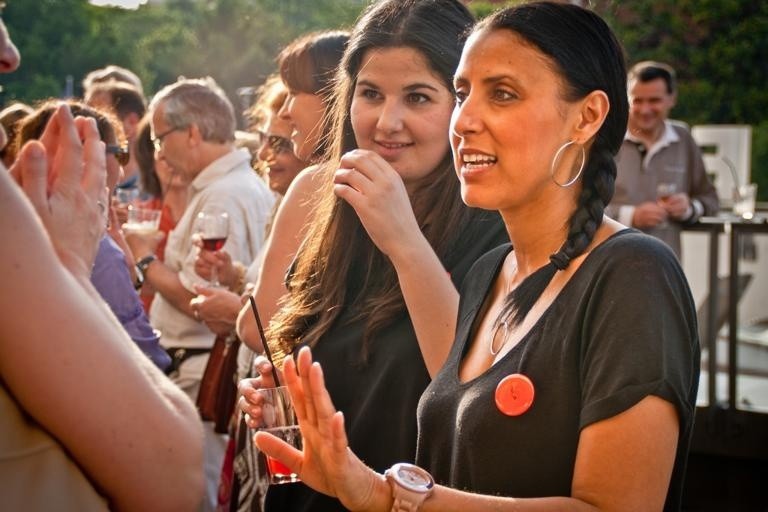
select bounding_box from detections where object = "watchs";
[386,459,437,510]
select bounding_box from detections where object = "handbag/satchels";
[195,332,241,436]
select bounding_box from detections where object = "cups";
[244,388,305,487]
[117,188,140,208]
[126,205,161,235]
[732,184,757,224]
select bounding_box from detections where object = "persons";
[237,1,701,510]
[599,61,719,266]
[238,0,508,511]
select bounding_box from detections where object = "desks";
[676,207,768,436]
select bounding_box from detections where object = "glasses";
[256,127,292,156]
[104,140,131,166]
[149,125,181,148]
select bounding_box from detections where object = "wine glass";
[194,208,232,295]
[658,182,679,233]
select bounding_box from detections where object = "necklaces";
[488,265,558,356]
